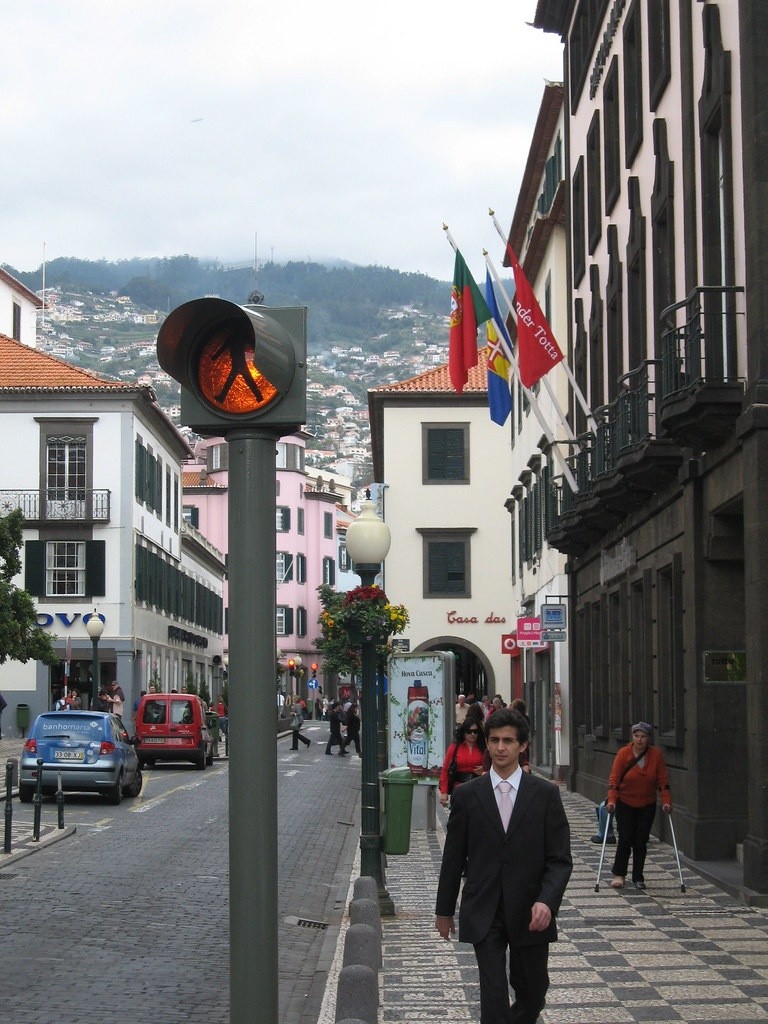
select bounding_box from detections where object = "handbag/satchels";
[288,714,300,731]
[318,703,323,712]
[439,759,458,795]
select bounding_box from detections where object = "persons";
[52,688,81,711]
[606,721,673,889]
[439,717,486,878]
[133,690,146,720]
[171,686,187,694]
[325,702,349,755]
[289,695,311,750]
[338,703,361,757]
[199,694,226,733]
[149,686,155,694]
[434,709,573,1024]
[591,801,616,843]
[90,681,125,720]
[455,690,530,774]
[315,697,353,721]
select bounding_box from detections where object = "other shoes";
[289,748,298,750]
[343,750,349,754]
[635,880,646,889]
[338,753,345,757]
[307,739,311,748]
[591,835,616,844]
[326,752,333,755]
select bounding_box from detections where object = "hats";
[632,722,651,734]
[112,681,118,685]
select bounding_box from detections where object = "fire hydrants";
[590,798,617,844]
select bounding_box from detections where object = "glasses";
[466,729,478,734]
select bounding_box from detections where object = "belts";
[456,772,478,783]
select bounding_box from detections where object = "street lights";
[87,608,105,711]
[345,489,396,917]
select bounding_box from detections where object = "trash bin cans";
[206,711,220,743]
[307,698,312,713]
[16,703,30,728]
[380,767,420,855]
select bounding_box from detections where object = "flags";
[449,250,493,395]
[507,243,564,390]
[485,267,513,426]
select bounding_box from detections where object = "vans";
[134,693,218,770]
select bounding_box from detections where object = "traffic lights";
[289,659,295,676]
[311,663,317,679]
[158,297,295,421]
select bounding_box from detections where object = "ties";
[498,782,514,834]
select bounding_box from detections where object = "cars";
[18,709,143,806]
